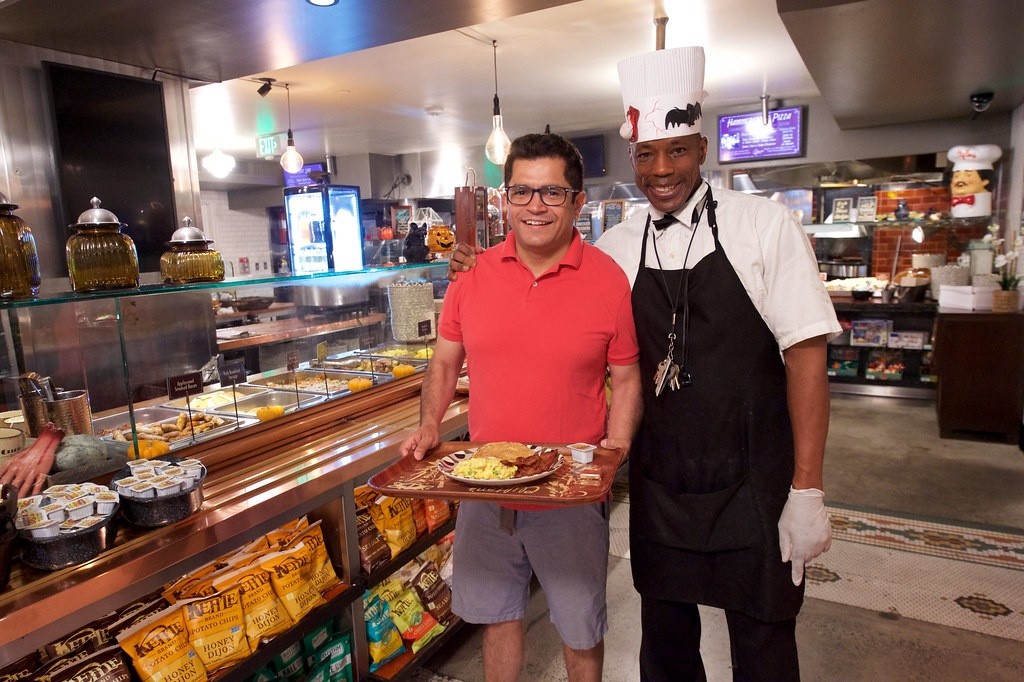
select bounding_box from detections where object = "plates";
[437,444,564,485]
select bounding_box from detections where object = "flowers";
[981,223,1024,291]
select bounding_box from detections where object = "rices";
[471,441,536,461]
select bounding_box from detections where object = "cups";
[18,388,94,438]
[881,291,894,302]
[0,410,28,458]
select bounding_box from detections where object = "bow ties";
[652,208,699,231]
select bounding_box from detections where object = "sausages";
[111,411,226,443]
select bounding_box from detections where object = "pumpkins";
[392,364,415,379]
[347,376,372,392]
[127,439,169,460]
[255,404,285,422]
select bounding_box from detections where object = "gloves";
[778,485,832,586]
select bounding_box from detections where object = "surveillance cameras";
[970,91,994,111]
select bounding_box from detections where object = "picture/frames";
[391,206,412,238]
[832,198,853,223]
[857,196,878,222]
[601,199,625,234]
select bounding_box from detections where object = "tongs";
[310,356,362,369]
[39,377,58,401]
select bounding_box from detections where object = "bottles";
[160,216,225,286]
[274,255,291,274]
[66,196,141,292]
[295,211,338,273]
[0,192,41,300]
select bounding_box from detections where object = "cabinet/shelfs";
[0,392,469,682]
[935,307,1024,445]
[827,297,938,399]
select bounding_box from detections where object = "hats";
[617,46,709,144]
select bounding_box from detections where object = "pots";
[0,484,18,595]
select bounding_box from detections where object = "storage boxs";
[829,318,937,385]
[939,284,990,312]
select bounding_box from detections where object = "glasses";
[505,186,580,206]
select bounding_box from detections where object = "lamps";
[912,225,944,244]
[257,78,276,97]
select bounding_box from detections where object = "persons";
[447,45,844,682]
[946,144,1002,218]
[400,135,644,682]
[0,423,65,500]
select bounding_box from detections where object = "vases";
[992,290,1019,313]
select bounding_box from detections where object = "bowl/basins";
[895,284,928,303]
[112,456,207,527]
[832,326,850,345]
[17,501,120,571]
[851,290,874,300]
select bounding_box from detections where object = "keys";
[653,358,679,396]
